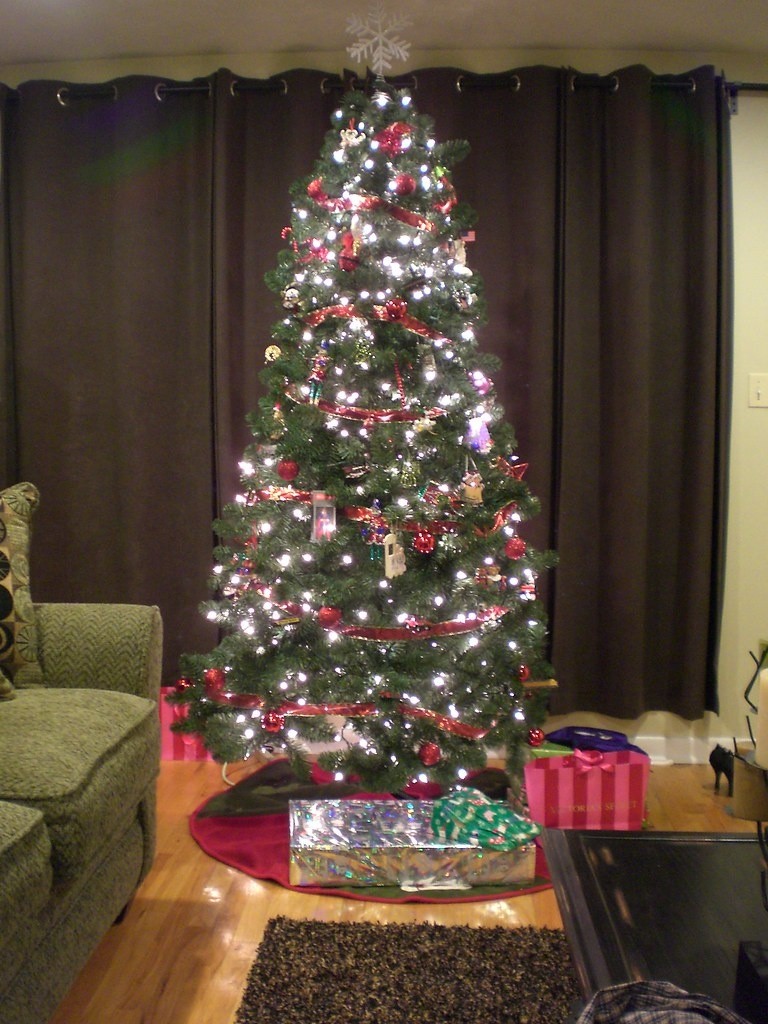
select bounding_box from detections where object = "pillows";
[0,481,46,689]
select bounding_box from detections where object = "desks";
[539,827,768,1024]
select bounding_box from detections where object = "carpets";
[188,760,654,904]
[235,913,587,1024]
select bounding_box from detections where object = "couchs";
[0,479,164,1024]
